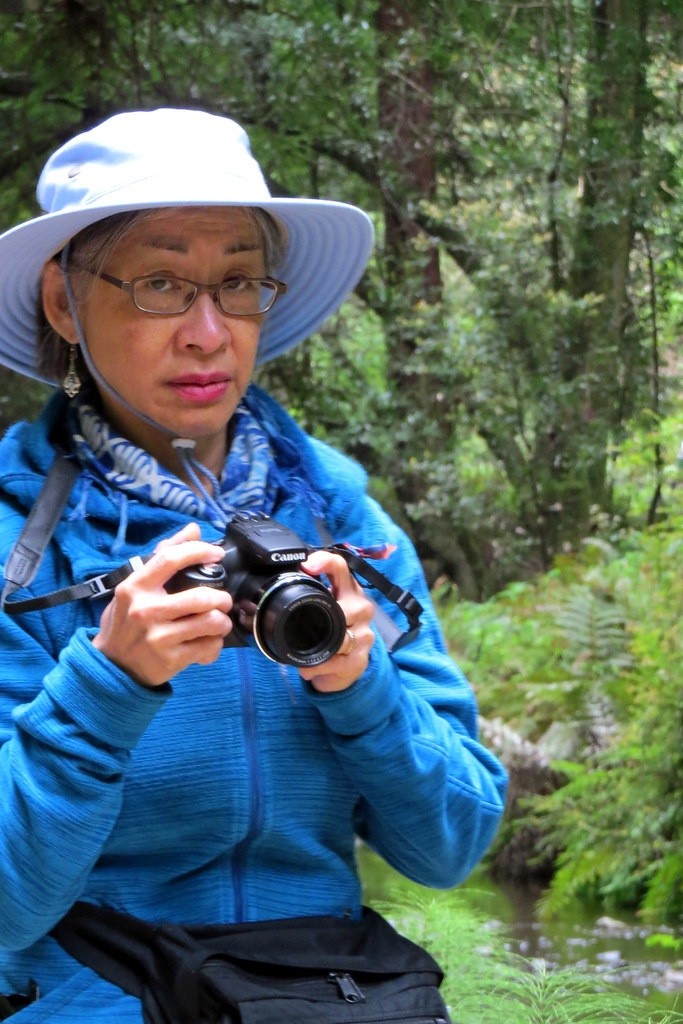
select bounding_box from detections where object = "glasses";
[53,241,291,321]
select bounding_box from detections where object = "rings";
[344,627,355,656]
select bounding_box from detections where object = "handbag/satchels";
[43,887,450,1024]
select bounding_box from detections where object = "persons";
[0,108,510,1023]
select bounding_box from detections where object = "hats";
[1,107,372,397]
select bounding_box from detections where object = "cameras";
[165,512,346,666]
